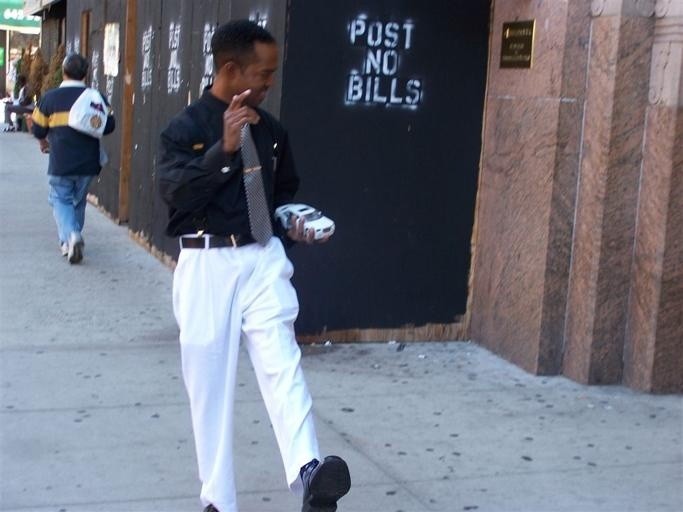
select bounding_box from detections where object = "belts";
[182,234,256,248]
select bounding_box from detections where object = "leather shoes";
[301,455,351,512]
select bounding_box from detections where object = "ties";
[240,122,273,247]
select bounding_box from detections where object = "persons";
[154,17,350,512]
[1,75,36,131]
[30,53,116,265]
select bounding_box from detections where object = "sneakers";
[67,230,84,264]
[60,241,69,256]
